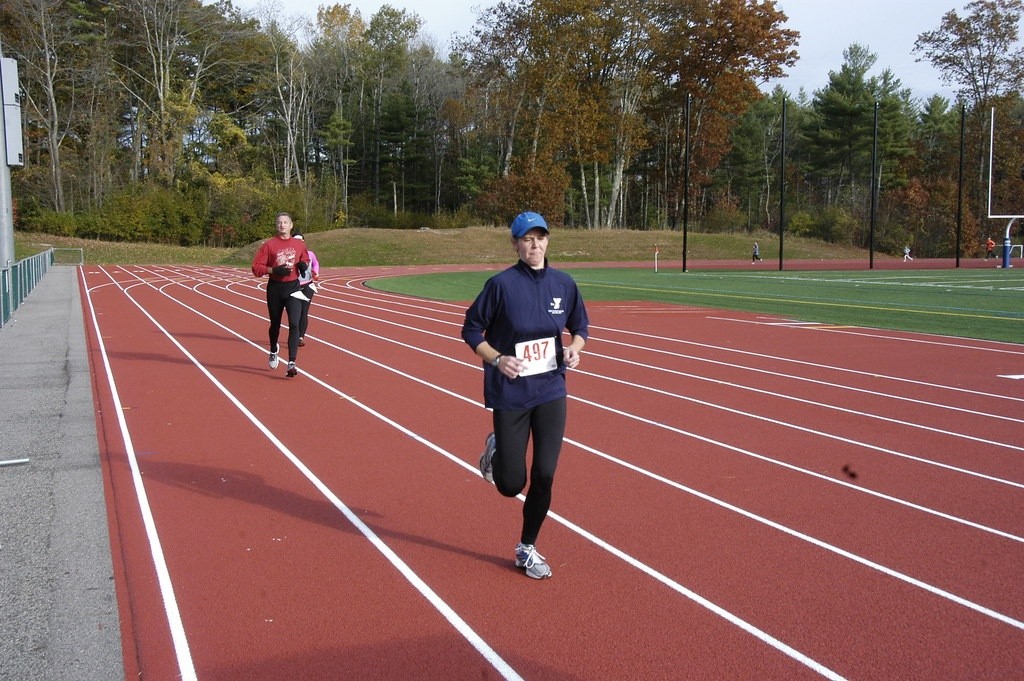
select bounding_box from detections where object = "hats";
[511,212,551,236]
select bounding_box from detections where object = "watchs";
[491,353,505,367]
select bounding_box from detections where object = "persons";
[902,244,914,263]
[752,241,762,264]
[251,213,311,378]
[984,237,1000,262]
[462,212,589,581]
[290,230,321,347]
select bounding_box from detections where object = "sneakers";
[515,542,553,580]
[269,343,280,370]
[298,338,305,347]
[479,433,496,483]
[285,368,297,377]
